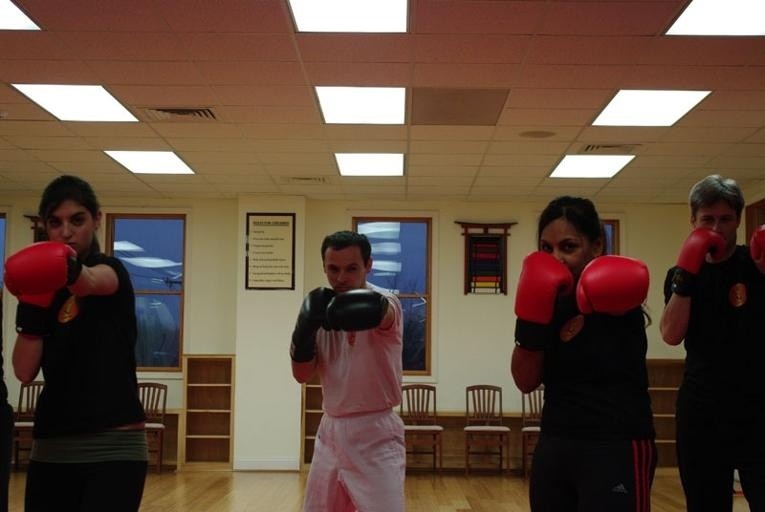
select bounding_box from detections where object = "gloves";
[15,288,72,338]
[669,225,727,299]
[326,287,389,332]
[3,238,84,297]
[574,254,651,337]
[290,286,338,364]
[738,223,765,273]
[514,249,576,352]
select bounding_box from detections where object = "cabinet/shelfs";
[181,351,237,471]
[302,379,329,463]
[644,359,691,469]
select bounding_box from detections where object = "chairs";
[137,381,166,473]
[12,383,45,465]
[518,383,549,476]
[463,384,510,477]
[399,385,444,477]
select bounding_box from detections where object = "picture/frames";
[244,211,296,290]
[597,218,619,260]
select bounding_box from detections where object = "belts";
[115,419,145,431]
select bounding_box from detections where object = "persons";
[659,174,765,512]
[290,230,407,512]
[4,174,148,512]
[511,195,657,512]
